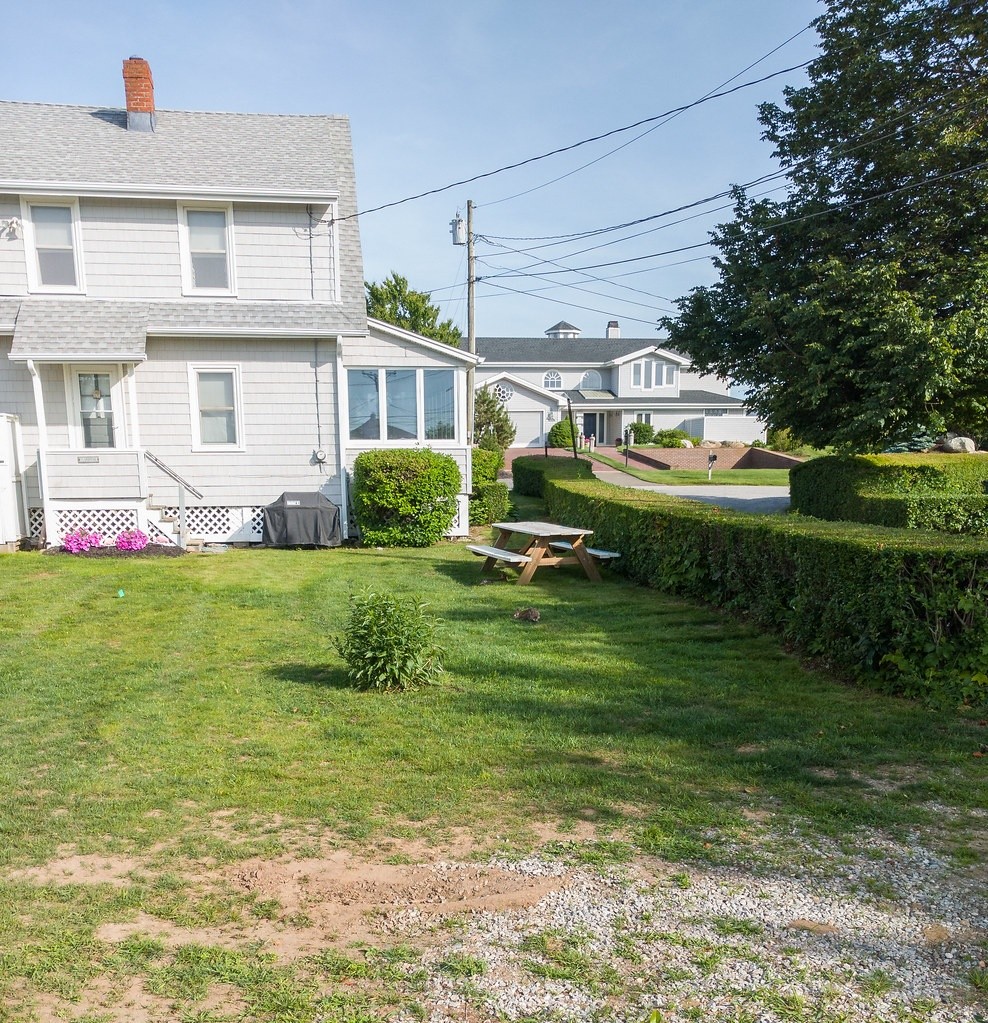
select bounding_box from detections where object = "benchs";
[465,545,533,566]
[548,541,622,563]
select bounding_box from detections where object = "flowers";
[585,439,589,443]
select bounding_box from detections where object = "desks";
[481,520,604,588]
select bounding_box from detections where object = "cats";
[513,608,542,622]
